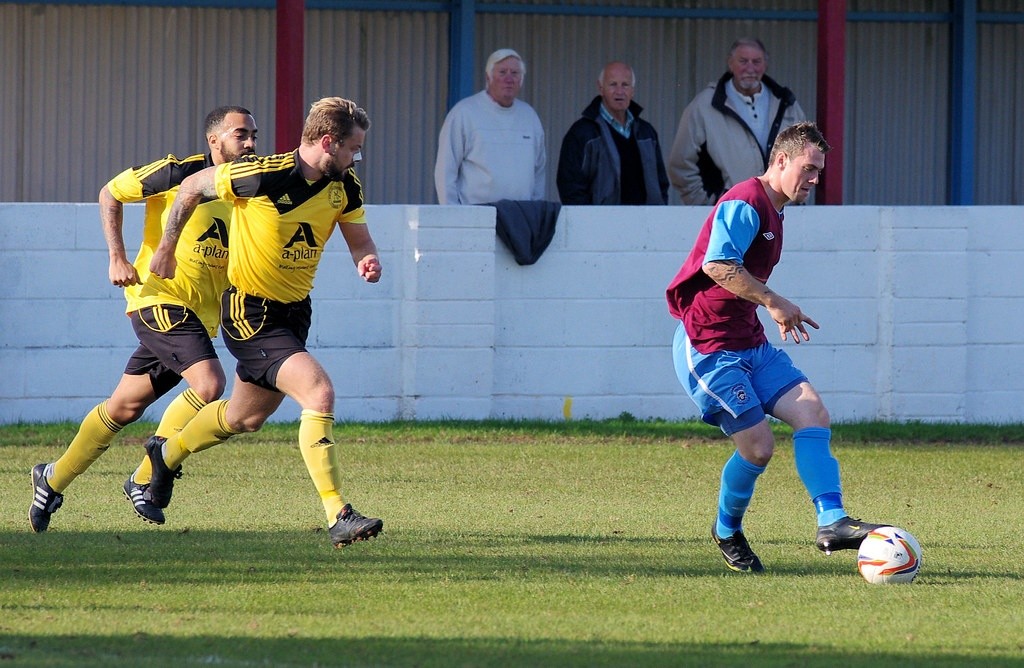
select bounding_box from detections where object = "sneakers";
[327,502,383,550]
[31,463,65,534]
[815,517,893,556]
[144,435,182,509]
[711,516,765,574]
[122,473,166,524]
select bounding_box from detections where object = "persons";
[435,49,546,205]
[666,122,892,573]
[145,97,384,548]
[669,37,806,205]
[557,61,671,205]
[28,106,259,533]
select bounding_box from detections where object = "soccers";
[857,526,923,586]
